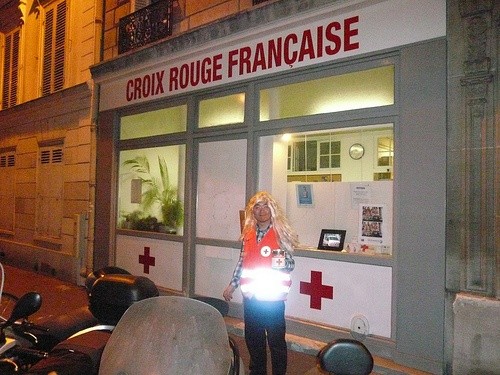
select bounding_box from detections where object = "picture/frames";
[295,183,313,208]
[317,229,346,251]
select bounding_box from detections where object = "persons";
[222,191,300,375]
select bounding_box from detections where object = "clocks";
[349,143,366,160]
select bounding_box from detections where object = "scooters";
[0,272,161,375]
[0,265,132,371]
